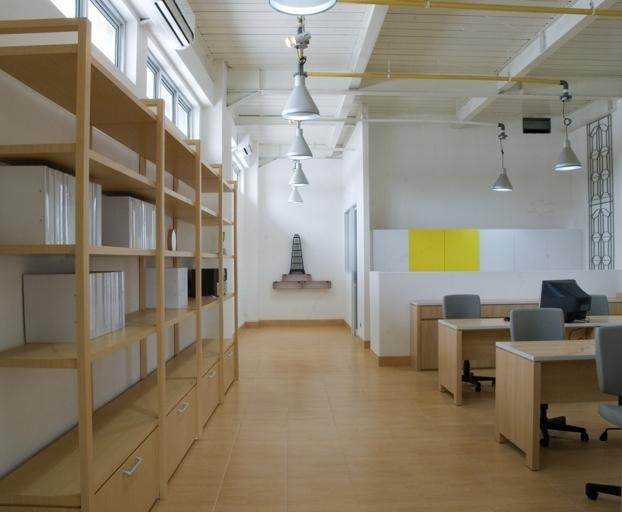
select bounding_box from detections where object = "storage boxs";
[188,268,227,298]
[105,196,158,248]
[143,268,190,309]
[22,271,126,344]
[0,166,103,247]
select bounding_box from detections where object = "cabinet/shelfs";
[1,16,240,512]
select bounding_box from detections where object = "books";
[23,271,125,342]
[145,267,188,308]
[102,197,157,249]
[2,166,102,246]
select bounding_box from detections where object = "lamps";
[554,101,583,171]
[269,1,336,207]
[488,123,515,193]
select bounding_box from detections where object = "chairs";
[586,482,622,500]
[593,324,622,441]
[588,294,610,316]
[441,293,496,393]
[509,308,589,449]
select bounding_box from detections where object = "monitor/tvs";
[540,279,591,322]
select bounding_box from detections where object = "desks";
[435,314,621,406]
[492,337,618,471]
[411,298,622,371]
